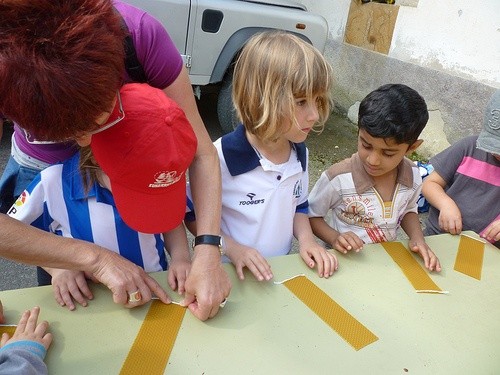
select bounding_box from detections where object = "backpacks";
[413,160,435,213]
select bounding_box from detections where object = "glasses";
[23,89,124,144]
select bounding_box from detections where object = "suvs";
[119,0,331,135]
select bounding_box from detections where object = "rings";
[219,297,227,307]
[128,289,142,302]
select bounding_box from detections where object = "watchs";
[192,234,225,256]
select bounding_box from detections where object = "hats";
[91,83,198,234]
[476,90,500,155]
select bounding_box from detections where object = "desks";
[0,230,499,375]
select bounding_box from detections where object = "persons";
[308,84,441,272]
[0,300,53,375]
[422,89,500,249]
[6,83,198,311]
[185,32,338,281]
[0,0,231,322]
